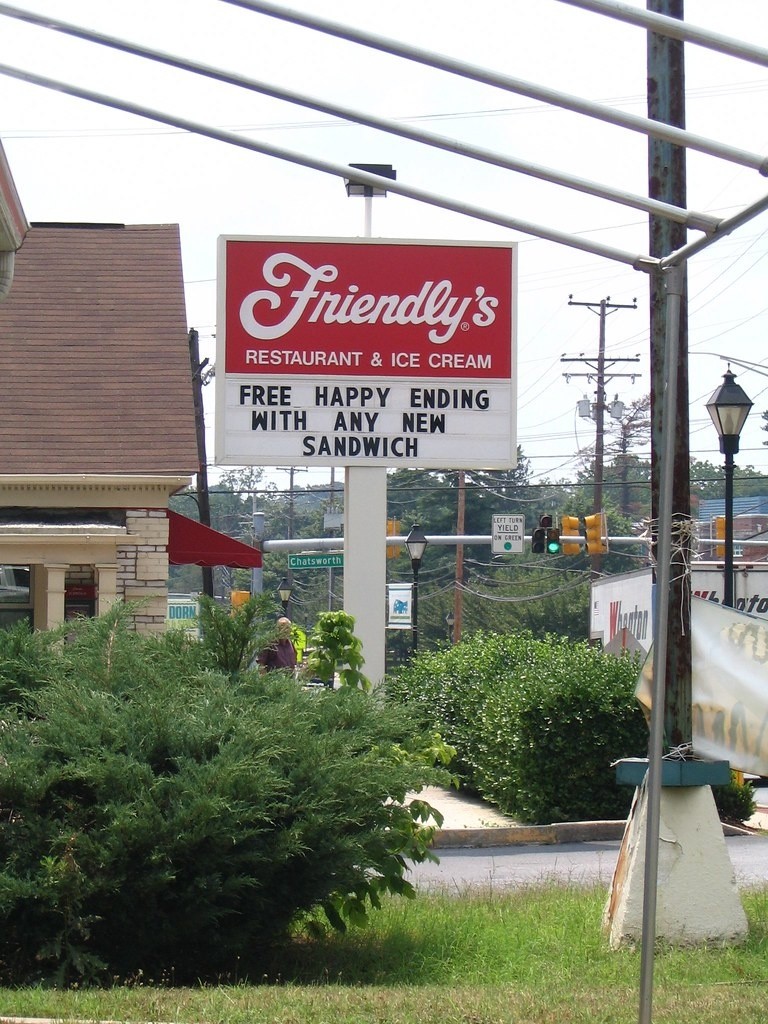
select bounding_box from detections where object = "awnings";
[166,507,261,571]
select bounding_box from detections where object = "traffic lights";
[547,528,562,554]
[540,513,554,527]
[530,527,546,555]
[561,513,583,555]
[583,513,604,554]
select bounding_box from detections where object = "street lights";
[704,360,754,604]
[405,521,428,655]
[277,575,294,618]
[304,606,311,634]
[444,610,454,648]
[345,161,403,237]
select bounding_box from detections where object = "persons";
[300,659,331,692]
[258,617,297,681]
